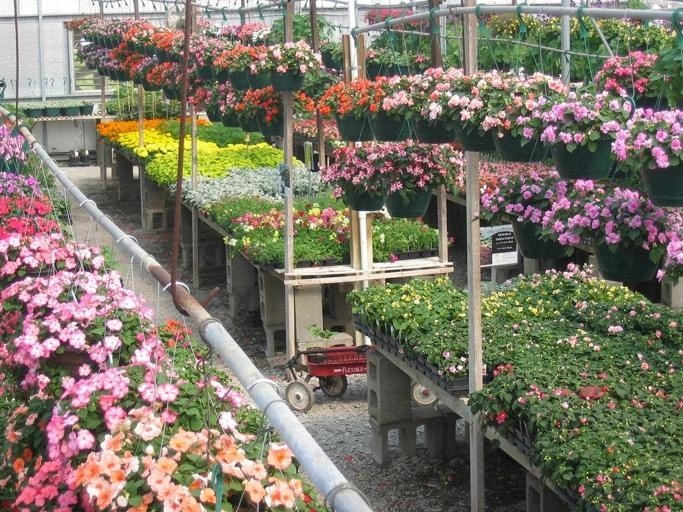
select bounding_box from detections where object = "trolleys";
[283,342,442,415]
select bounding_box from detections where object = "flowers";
[1,0,683,512]
